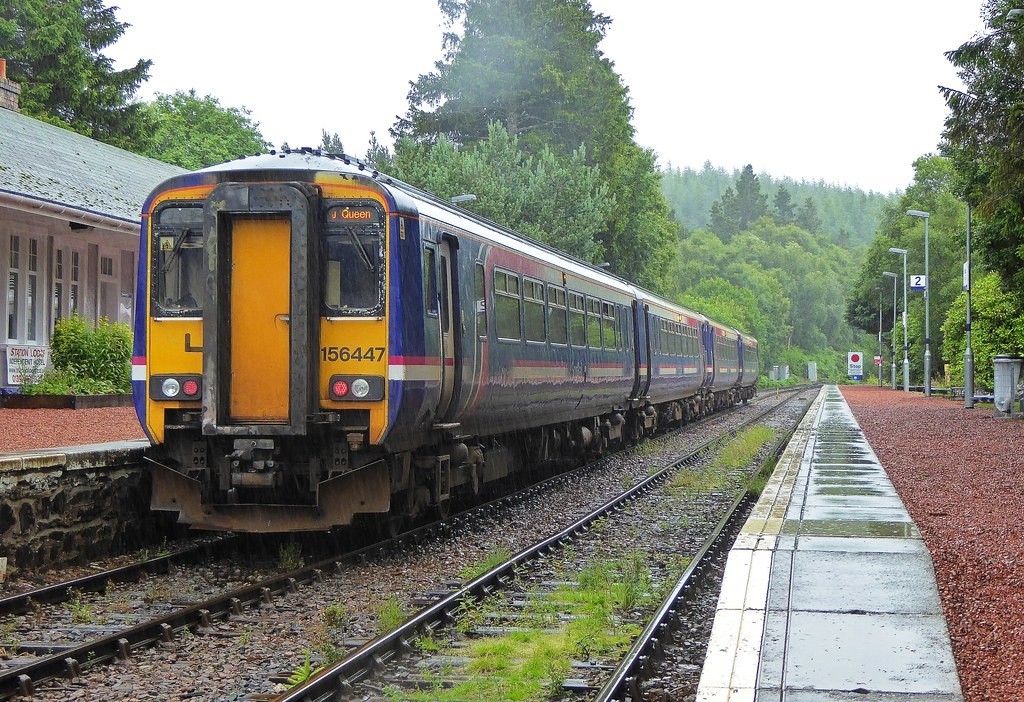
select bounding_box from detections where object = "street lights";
[905,210,932,398]
[888,248,910,393]
[882,271,897,391]
[875,286,883,387]
[957,195,974,409]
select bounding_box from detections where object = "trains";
[130,148,761,552]
